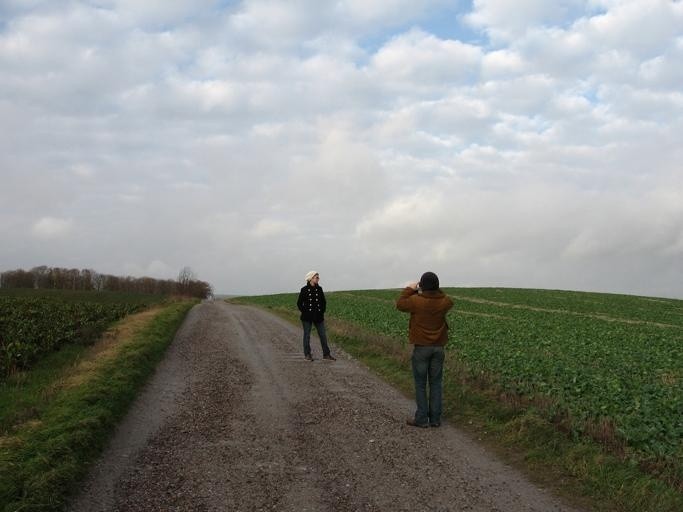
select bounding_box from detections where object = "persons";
[397,271,454,427]
[297,271,337,361]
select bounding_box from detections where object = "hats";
[305,271,318,282]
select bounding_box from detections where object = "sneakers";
[406,415,442,429]
[304,354,312,362]
[322,356,337,361]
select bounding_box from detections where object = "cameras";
[415,282,423,290]
